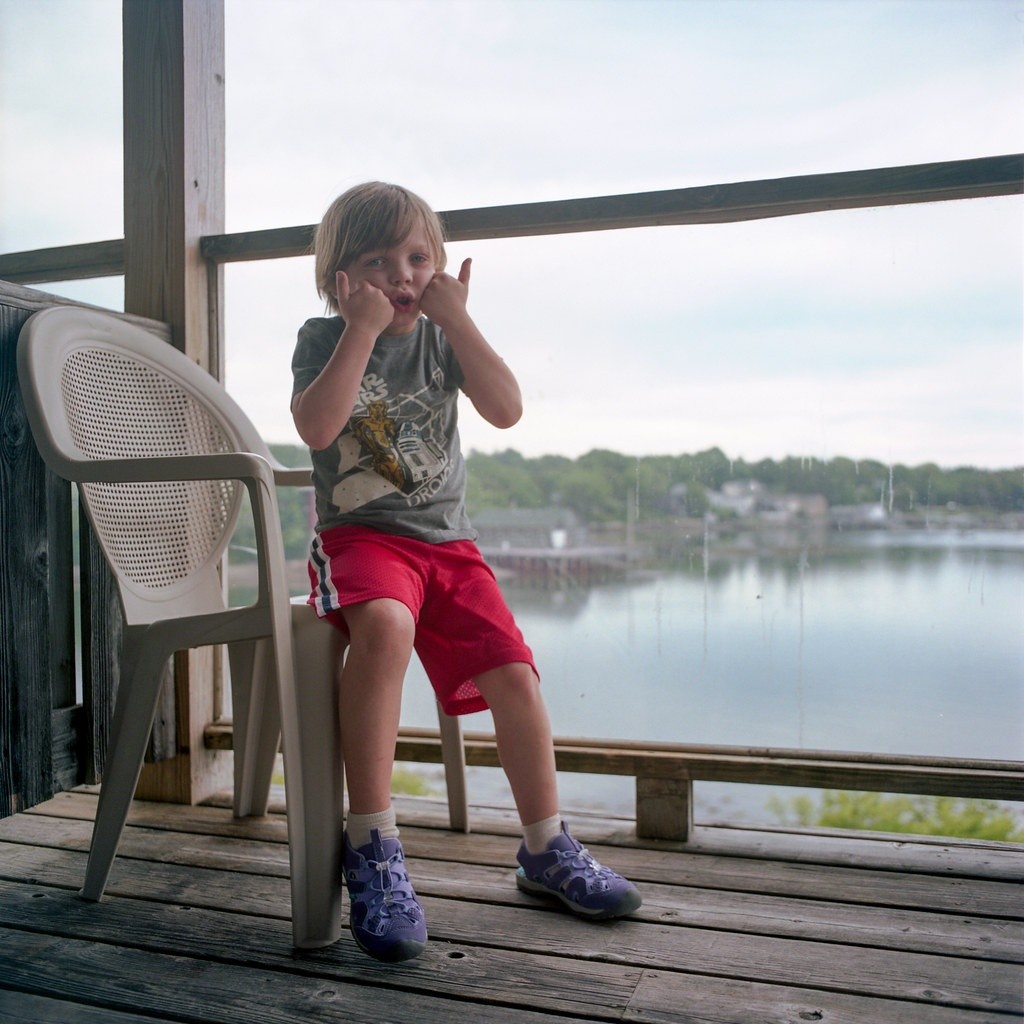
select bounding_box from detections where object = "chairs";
[16,304,472,949]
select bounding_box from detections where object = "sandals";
[341,827,427,960]
[517,819,643,918]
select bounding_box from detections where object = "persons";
[291,181,643,962]
